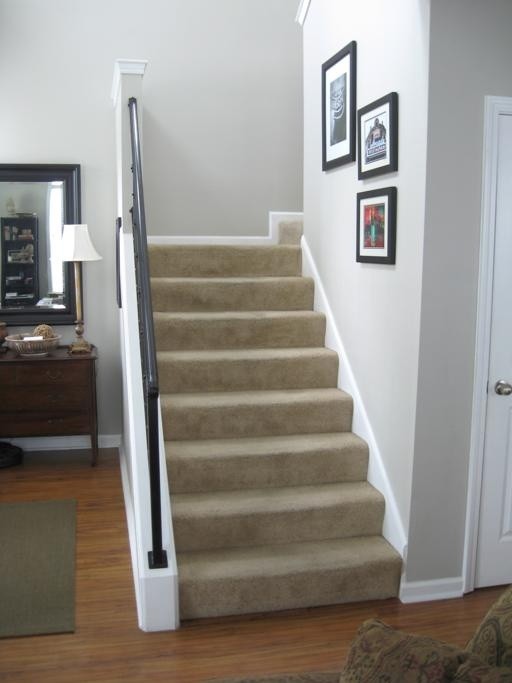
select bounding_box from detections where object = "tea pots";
[0,162,83,325]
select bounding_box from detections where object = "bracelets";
[4,332,62,358]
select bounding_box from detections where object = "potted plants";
[318,36,399,266]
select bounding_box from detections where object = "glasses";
[49,224,103,356]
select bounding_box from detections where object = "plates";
[0,344,98,467]
[0,214,39,307]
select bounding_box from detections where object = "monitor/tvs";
[8,276,22,280]
[5,292,34,299]
[5,225,35,240]
[36,299,53,308]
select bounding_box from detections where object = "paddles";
[0,496,77,639]
[143,221,403,623]
